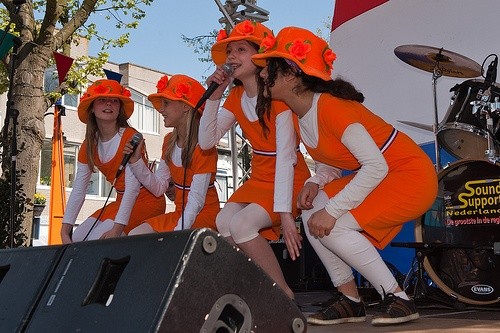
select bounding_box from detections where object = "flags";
[48,106,66,246]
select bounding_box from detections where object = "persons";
[59,79,166,243]
[198,20,311,298]
[251,27,439,325]
[122,75,220,236]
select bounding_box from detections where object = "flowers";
[259,30,278,53]
[285,37,312,64]
[123,87,132,99]
[155,74,169,94]
[322,46,338,71]
[173,80,194,99]
[93,82,112,94]
[235,19,257,36]
[215,28,229,40]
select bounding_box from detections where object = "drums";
[414,157,500,306]
[436,79,500,160]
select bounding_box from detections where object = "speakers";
[19,227,309,333]
[268,240,310,288]
[0,244,67,333]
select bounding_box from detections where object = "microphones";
[196,63,234,109]
[428,296,466,311]
[494,243,500,255]
[490,56,498,84]
[117,133,142,176]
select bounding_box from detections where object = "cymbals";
[396,120,433,132]
[394,45,485,79]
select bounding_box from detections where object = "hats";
[210,19,278,69]
[251,26,337,82]
[146,74,209,114]
[77,79,135,125]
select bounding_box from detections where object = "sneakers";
[306,289,367,325]
[371,284,422,326]
